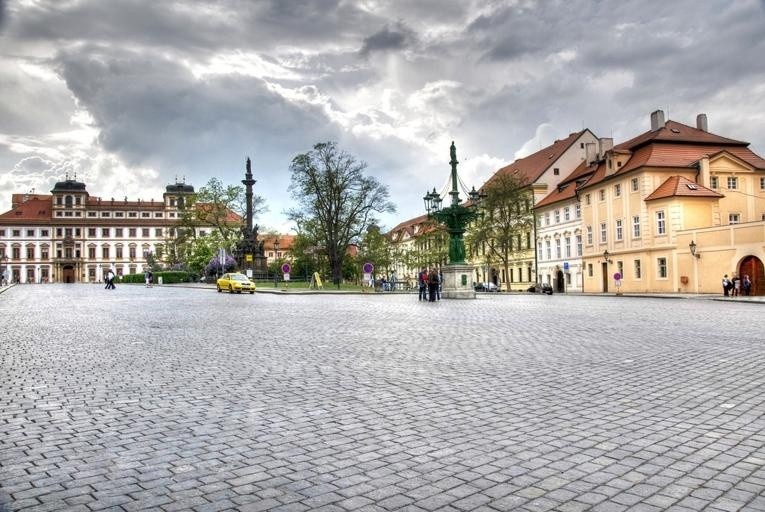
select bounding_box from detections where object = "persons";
[118,269,124,284]
[143,269,154,289]
[105,268,115,290]
[367,269,411,293]
[417,267,441,301]
[528,283,553,295]
[721,270,756,298]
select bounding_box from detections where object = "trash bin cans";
[158,276,163,284]
[383,282,391,291]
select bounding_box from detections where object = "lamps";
[689,241,700,258]
[604,250,613,263]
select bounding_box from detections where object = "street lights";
[4,255,9,286]
[99,264,102,283]
[273,238,280,289]
[423,140,488,267]
[37,267,40,284]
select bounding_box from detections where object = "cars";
[476,281,499,293]
[216,272,257,295]
[526,283,554,295]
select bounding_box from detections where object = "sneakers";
[432,300,436,302]
[419,299,422,301]
[437,299,440,300]
[429,300,432,302]
[424,299,428,301]
